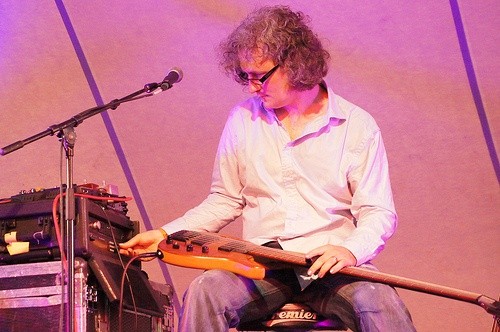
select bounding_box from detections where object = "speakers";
[0,257,174,332]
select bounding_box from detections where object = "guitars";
[157,229,500,317]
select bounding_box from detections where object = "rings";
[331,256,338,263]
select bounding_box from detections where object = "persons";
[119,4,418,332]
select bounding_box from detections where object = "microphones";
[161,67,183,91]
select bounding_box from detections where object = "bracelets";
[158,228,168,240]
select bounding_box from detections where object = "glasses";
[238,62,283,85]
